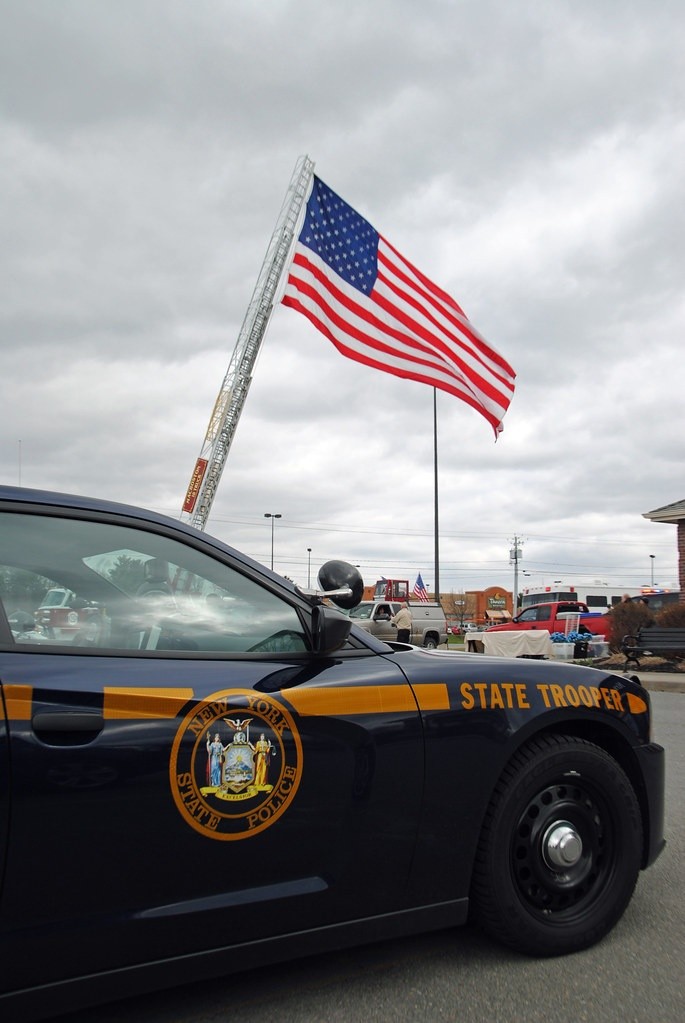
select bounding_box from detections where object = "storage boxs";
[590,641,610,657]
[553,642,575,660]
[592,634,606,642]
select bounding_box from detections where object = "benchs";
[621,625,685,673]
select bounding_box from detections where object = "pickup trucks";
[487,601,612,635]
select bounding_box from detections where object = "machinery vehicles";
[372,579,409,602]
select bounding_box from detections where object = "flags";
[277,174,517,444]
[413,574,429,602]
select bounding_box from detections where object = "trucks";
[347,601,447,650]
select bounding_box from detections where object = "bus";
[521,586,643,616]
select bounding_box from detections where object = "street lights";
[265,513,282,571]
[308,549,311,589]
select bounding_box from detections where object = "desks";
[464,630,554,661]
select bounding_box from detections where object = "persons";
[619,594,649,609]
[377,607,389,619]
[390,602,412,643]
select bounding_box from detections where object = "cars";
[0,485,667,1023]
